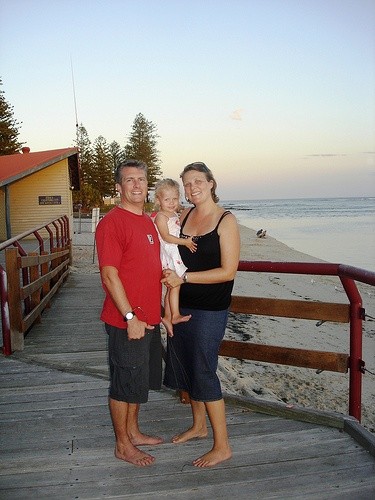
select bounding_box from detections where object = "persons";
[90,158,164,468]
[256,228,264,237]
[153,176,193,338]
[159,161,241,469]
[123,311,135,322]
[261,230,267,237]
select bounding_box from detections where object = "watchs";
[182,271,188,283]
